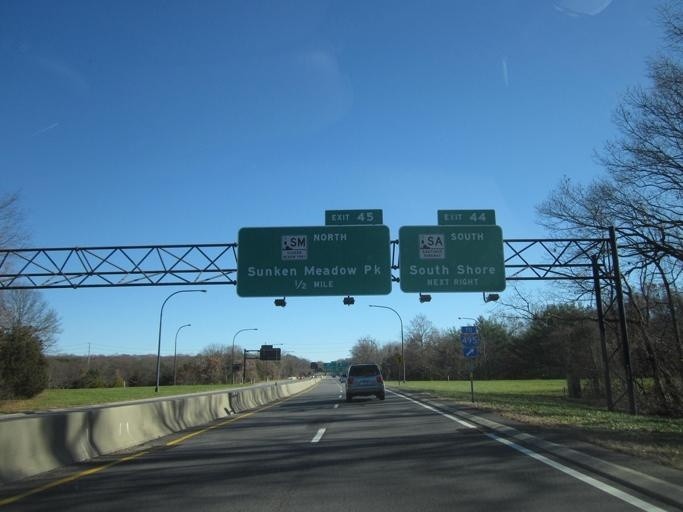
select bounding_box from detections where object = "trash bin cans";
[567,377,581,398]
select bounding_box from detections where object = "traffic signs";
[400,225,506,292]
[460,326,481,358]
[437,209,496,227]
[325,209,383,226]
[238,224,392,297]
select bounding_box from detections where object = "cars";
[340,374,347,383]
[345,363,386,402]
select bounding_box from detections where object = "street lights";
[173,324,191,386]
[359,339,378,364]
[369,305,406,384]
[230,328,295,383]
[155,289,207,392]
